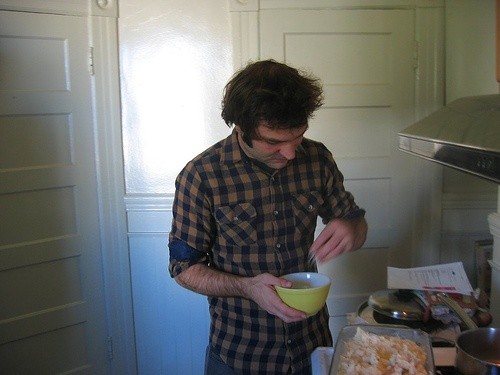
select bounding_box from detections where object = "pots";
[435,291,500,375]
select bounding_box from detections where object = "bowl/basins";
[274,272,332,316]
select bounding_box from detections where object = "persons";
[168,58,368,375]
